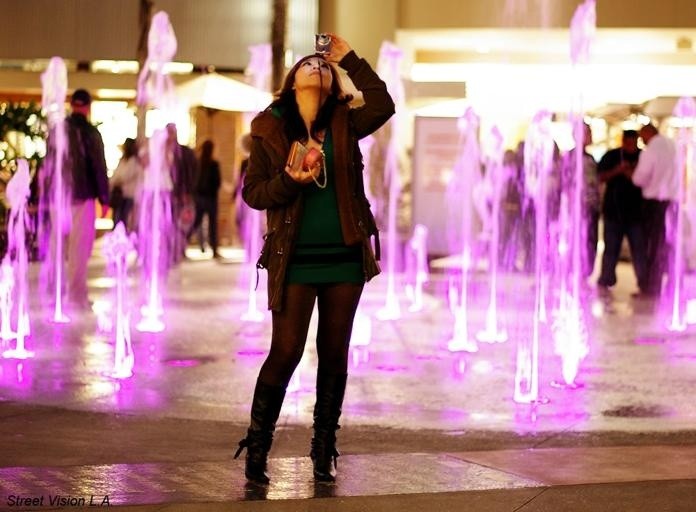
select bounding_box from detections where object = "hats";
[72,89,90,106]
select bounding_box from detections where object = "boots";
[244,376,287,485]
[310,368,348,480]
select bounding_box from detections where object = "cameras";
[316,34,330,53]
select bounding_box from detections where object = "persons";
[109,123,221,258]
[498,124,676,299]
[45,89,109,311]
[241,32,395,487]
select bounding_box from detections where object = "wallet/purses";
[286,139,309,171]
